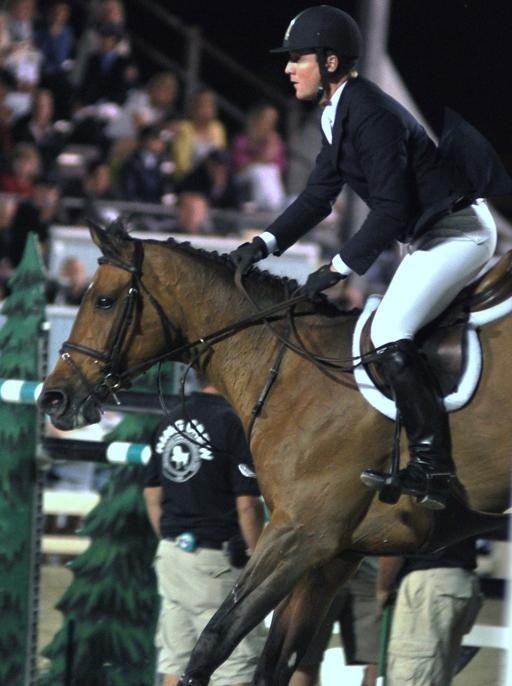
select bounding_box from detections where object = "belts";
[413,192,478,241]
[171,533,222,549]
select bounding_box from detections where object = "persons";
[213,4,498,516]
[284,551,383,686]
[139,378,272,685]
[2,1,296,307]
[381,528,486,686]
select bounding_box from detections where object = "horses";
[38,211,512,686]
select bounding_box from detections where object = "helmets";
[269,5,363,80]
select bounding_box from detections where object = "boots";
[360,337,462,499]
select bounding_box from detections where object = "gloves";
[304,263,347,299]
[229,237,268,275]
